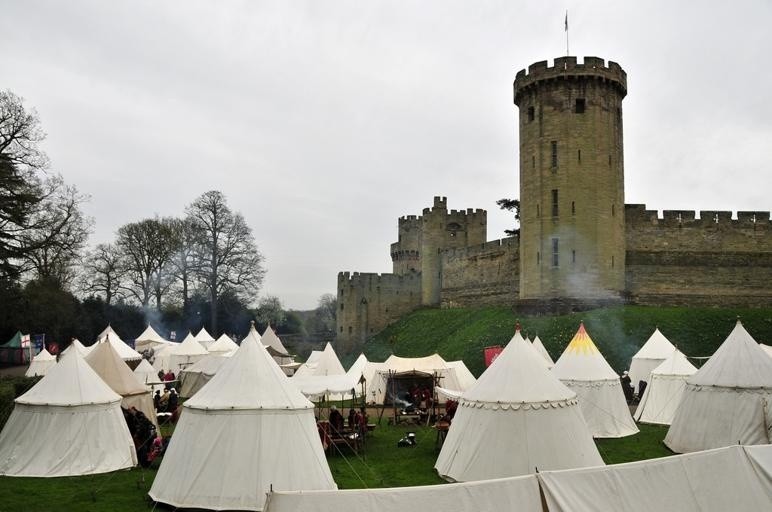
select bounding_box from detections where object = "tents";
[2,317,772,510]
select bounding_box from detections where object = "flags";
[563,12,568,31]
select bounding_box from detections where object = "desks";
[387,414,422,425]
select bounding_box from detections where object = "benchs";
[327,423,376,457]
